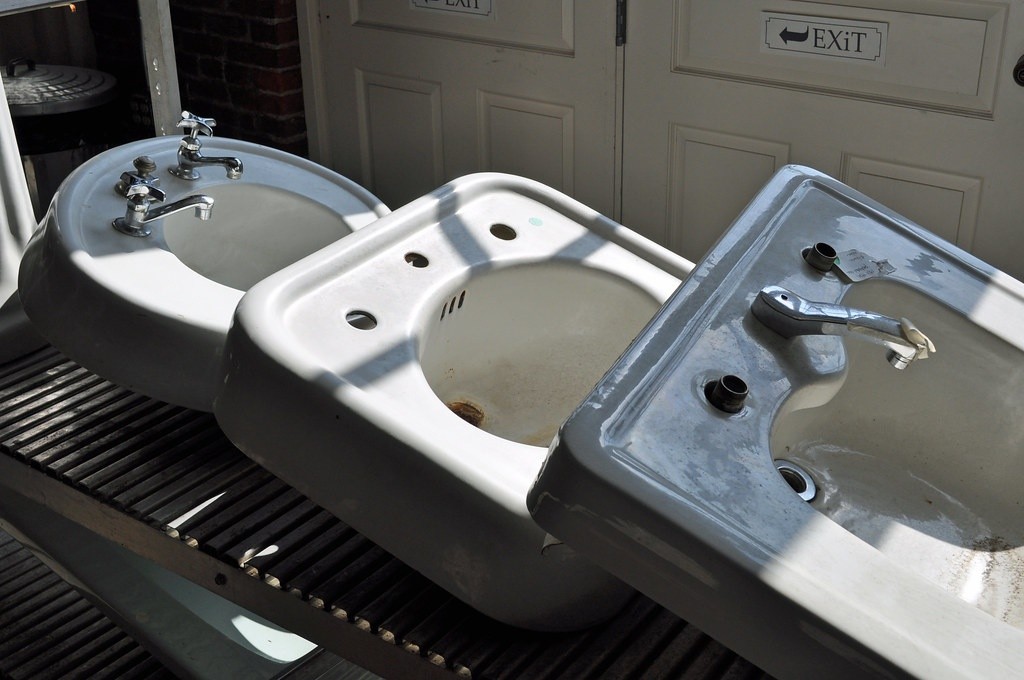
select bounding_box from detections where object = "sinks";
[524,164,1019,677]
[209,170,695,634]
[14,131,393,415]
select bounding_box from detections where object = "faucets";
[109,170,216,239]
[167,111,244,181]
[746,284,924,372]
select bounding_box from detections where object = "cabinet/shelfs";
[295,0,1024,281]
